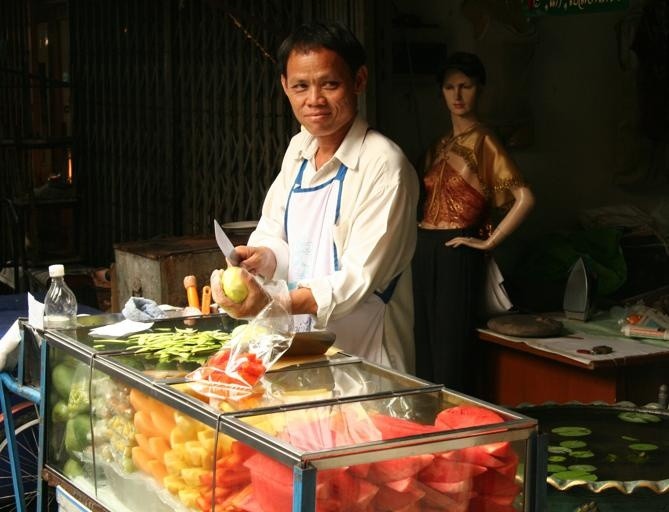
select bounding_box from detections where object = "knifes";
[213,219,242,265]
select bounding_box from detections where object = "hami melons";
[127,382,334,509]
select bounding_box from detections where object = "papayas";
[222,267,248,303]
[49,356,201,479]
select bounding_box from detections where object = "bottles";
[43,264,78,331]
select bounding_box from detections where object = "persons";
[410,51,536,407]
[210,37,422,422]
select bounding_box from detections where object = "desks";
[476,326,669,408]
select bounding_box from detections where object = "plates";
[221,221,259,236]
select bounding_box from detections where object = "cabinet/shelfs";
[19,308,551,512]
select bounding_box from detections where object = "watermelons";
[231,405,522,512]
